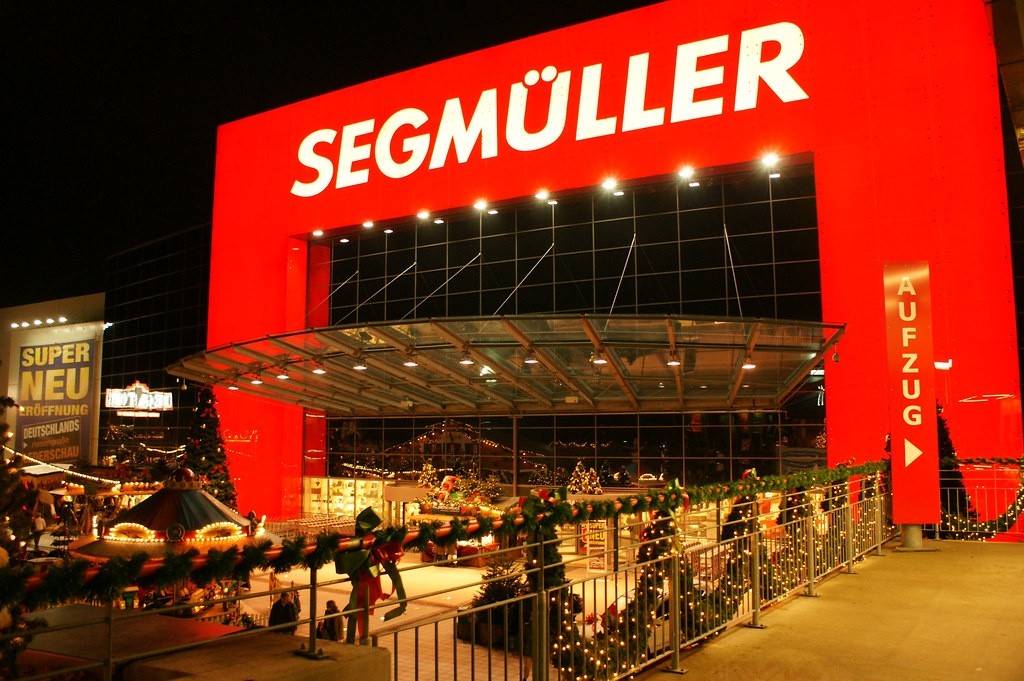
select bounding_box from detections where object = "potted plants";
[453,554,582,657]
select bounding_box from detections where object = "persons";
[317,600,345,641]
[269,590,299,634]
[32,513,47,550]
